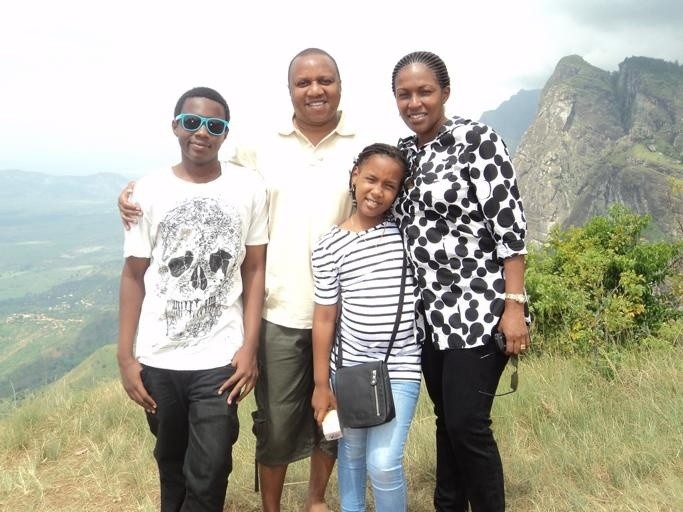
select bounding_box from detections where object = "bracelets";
[503,293,527,307]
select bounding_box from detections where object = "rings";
[519,342,526,350]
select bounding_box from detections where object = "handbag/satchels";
[334,361,395,429]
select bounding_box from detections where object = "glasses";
[479,346,518,396]
[176,113,228,137]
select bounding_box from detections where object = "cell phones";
[494,332,507,352]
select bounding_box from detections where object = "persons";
[114,88,270,512]
[115,48,367,511]
[390,49,532,511]
[305,142,426,511]
